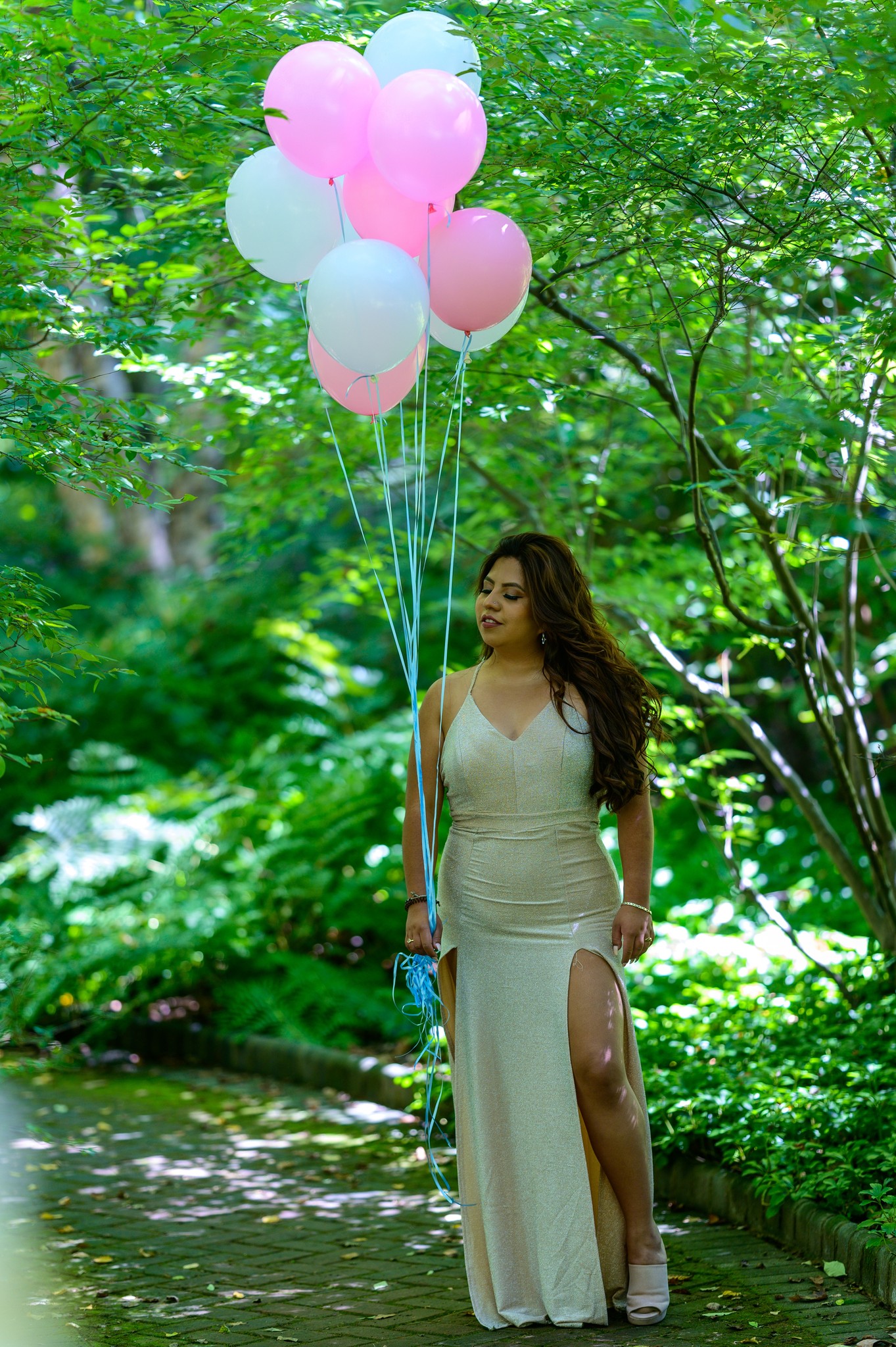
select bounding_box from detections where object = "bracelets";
[621,902,652,916]
[404,891,440,912]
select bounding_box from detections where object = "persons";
[401,531,671,1331]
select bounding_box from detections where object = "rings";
[644,937,652,942]
[407,939,413,943]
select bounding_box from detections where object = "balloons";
[367,68,488,212]
[305,238,431,383]
[337,173,362,244]
[308,325,427,424]
[224,144,345,292]
[263,41,382,187]
[413,256,530,365]
[343,156,456,258]
[418,206,533,338]
[362,11,482,98]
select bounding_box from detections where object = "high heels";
[627,1263,670,1325]
